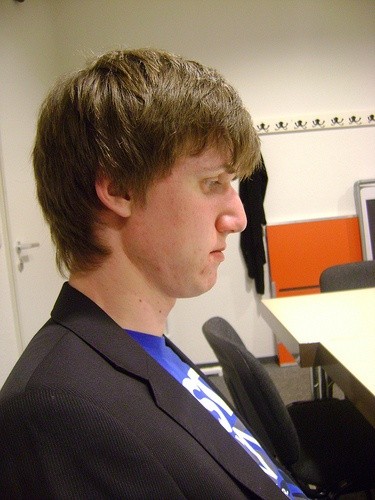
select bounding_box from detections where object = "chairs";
[199,317,375,500]
[319,257,374,292]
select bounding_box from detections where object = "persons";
[1,50,312,500]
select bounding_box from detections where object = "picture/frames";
[354,178,374,262]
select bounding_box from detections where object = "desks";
[256,282,374,429]
